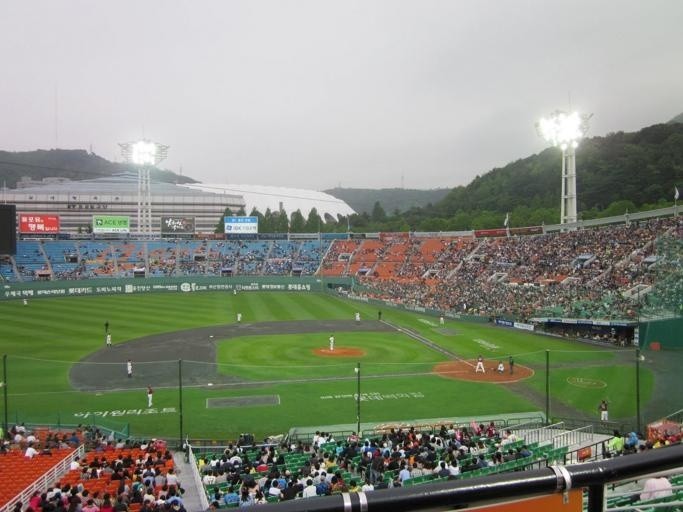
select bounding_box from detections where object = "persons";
[0,217,683,512]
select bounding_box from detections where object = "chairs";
[191,428,570,511]
[0,427,176,512]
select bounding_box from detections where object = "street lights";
[118,140,168,239]
[537,111,589,233]
